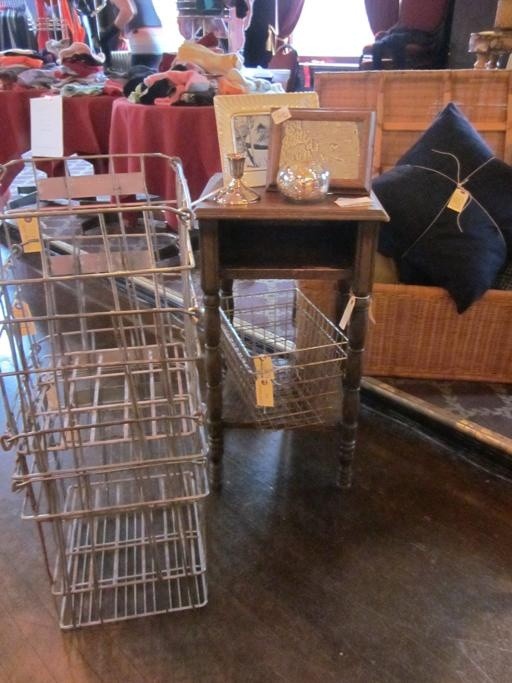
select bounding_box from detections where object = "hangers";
[0,0,25,10]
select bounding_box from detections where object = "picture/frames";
[213,92,320,186]
[266,106,376,198]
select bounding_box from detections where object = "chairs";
[267,45,298,92]
[358,0,456,70]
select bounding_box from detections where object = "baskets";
[219,287,350,432]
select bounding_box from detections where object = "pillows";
[370,102,512,314]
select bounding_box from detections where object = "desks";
[190,173,392,492]
[0,76,222,231]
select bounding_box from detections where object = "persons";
[91,0,163,71]
